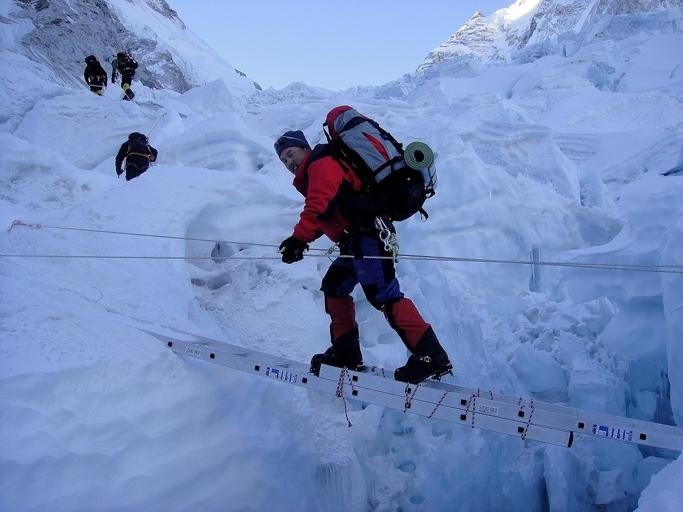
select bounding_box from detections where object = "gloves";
[279,237,308,263]
[86,81,89,85]
[104,81,108,87]
[116,169,123,175]
[112,75,118,84]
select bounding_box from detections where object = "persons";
[111,53,138,101]
[84,55,107,96]
[274,130,453,385]
[116,133,158,181]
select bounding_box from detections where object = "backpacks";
[117,52,138,69]
[85,55,98,71]
[322,105,435,222]
[128,132,149,155]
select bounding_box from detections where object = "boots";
[123,89,135,101]
[394,326,453,383]
[311,326,362,376]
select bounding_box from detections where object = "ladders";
[101,310,683,452]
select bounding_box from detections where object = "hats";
[274,130,309,157]
